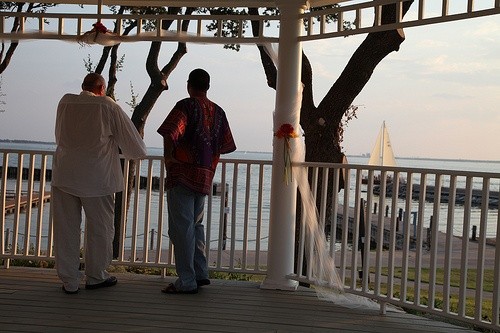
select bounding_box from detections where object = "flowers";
[273,123,299,188]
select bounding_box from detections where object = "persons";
[157,68,237,295]
[50,72,153,295]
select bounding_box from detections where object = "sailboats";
[362,120,406,184]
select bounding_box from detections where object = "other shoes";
[63,283,81,294]
[84,276,118,289]
[195,277,211,285]
[161,282,200,294]
